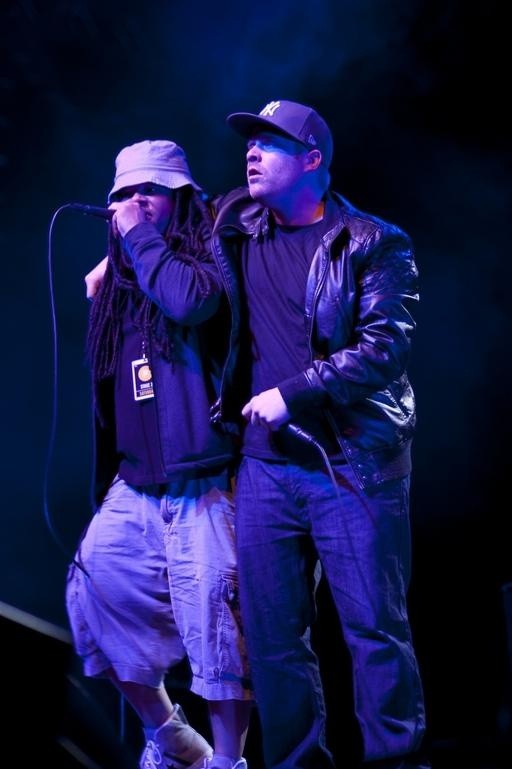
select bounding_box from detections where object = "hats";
[226,101,335,164]
[108,139,204,195]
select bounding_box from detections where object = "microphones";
[73,203,115,219]
[282,422,317,448]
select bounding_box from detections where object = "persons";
[65,141,259,769]
[84,98,429,769]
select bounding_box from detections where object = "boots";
[204,757,249,769]
[138,703,214,769]
